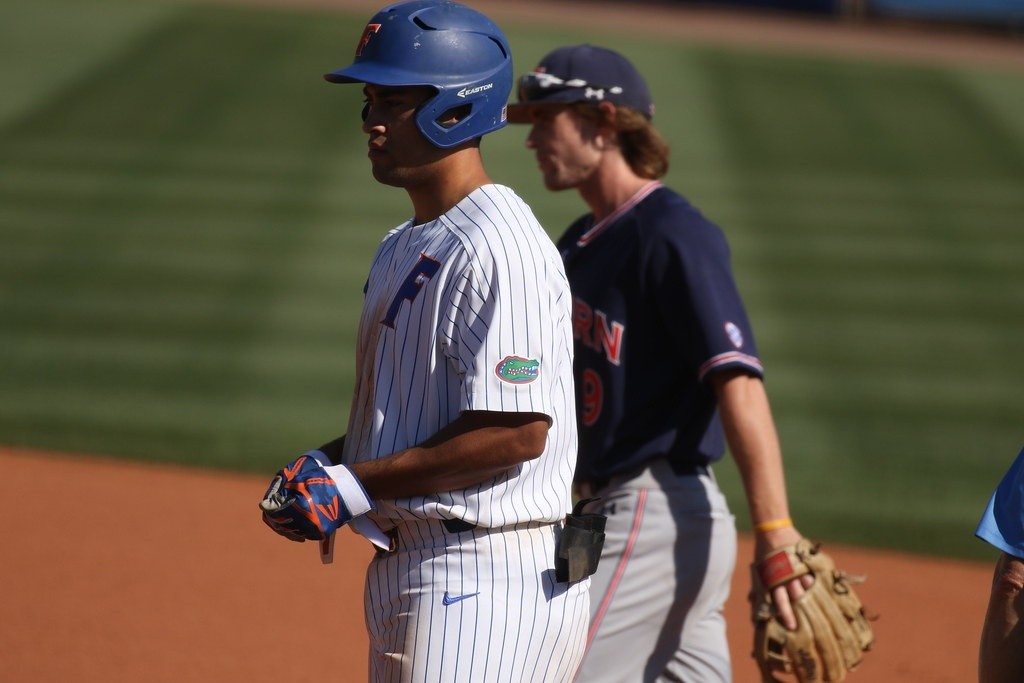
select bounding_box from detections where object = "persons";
[259,0,591,683]
[507,44,874,683]
[975,448,1024,683]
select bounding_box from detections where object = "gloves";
[258,449,392,563]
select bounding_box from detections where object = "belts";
[365,514,478,556]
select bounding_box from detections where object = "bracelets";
[754,519,794,532]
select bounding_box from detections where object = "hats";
[508,43,655,123]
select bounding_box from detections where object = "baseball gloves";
[749,539,874,683]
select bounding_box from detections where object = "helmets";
[323,0,514,149]
